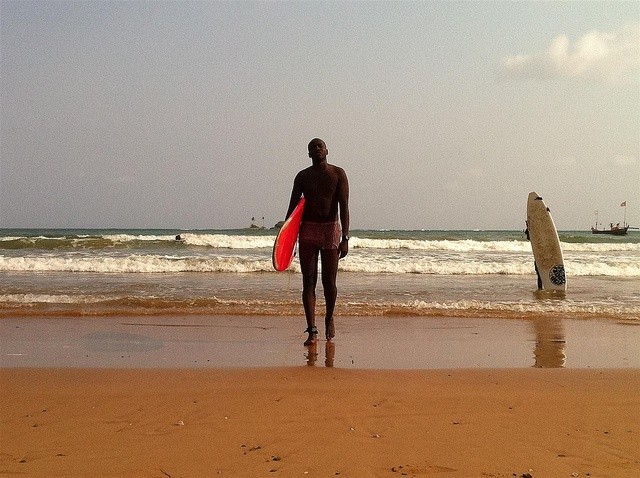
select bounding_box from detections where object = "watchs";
[342,235,350,241]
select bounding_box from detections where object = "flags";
[621,201,626,206]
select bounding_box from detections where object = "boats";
[591,200,628,236]
[243,216,271,231]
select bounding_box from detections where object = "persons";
[285,138,350,344]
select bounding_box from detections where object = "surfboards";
[272,197,305,271]
[527,192,567,291]
